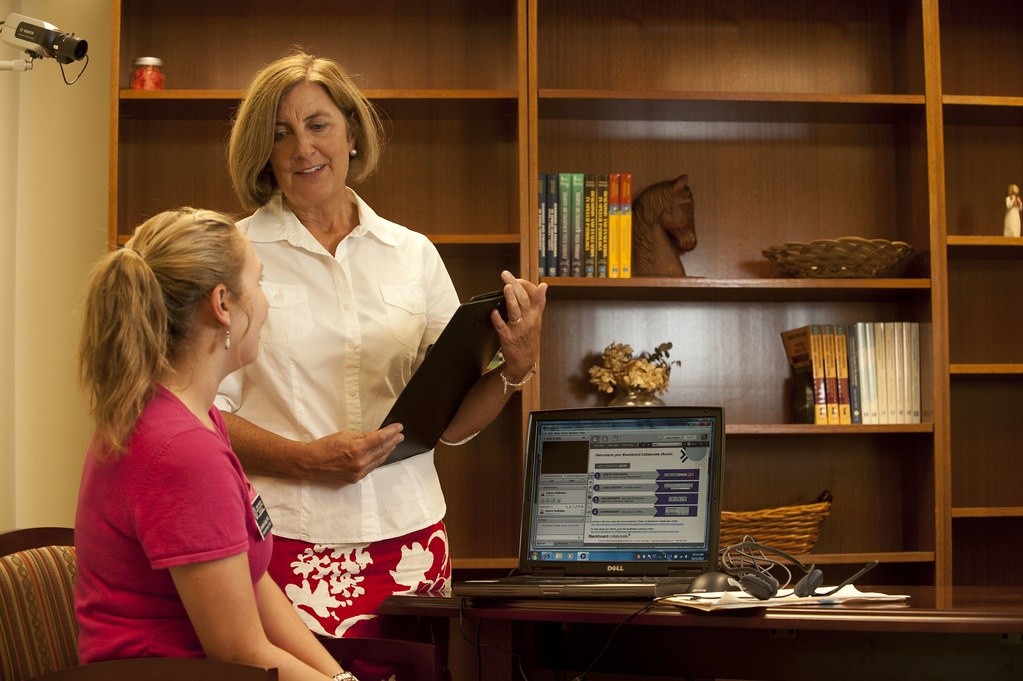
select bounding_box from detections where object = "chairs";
[0,526,279,681]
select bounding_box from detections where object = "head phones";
[722,541,825,600]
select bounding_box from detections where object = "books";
[537,172,632,278]
[781,323,921,425]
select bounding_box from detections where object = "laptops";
[451,405,727,599]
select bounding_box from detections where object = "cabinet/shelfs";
[114,0,1022,680]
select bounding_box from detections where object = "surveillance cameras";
[0,11,89,72]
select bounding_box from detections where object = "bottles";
[128,57,166,92]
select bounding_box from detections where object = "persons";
[1004,184,1023,237]
[213,55,548,681]
[73,205,345,681]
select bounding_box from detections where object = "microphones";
[816,559,881,598]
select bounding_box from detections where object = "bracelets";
[500,363,536,394]
[333,671,359,681]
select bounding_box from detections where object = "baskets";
[761,237,909,278]
[718,491,834,554]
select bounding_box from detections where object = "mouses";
[687,572,747,593]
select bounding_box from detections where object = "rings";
[510,318,521,324]
